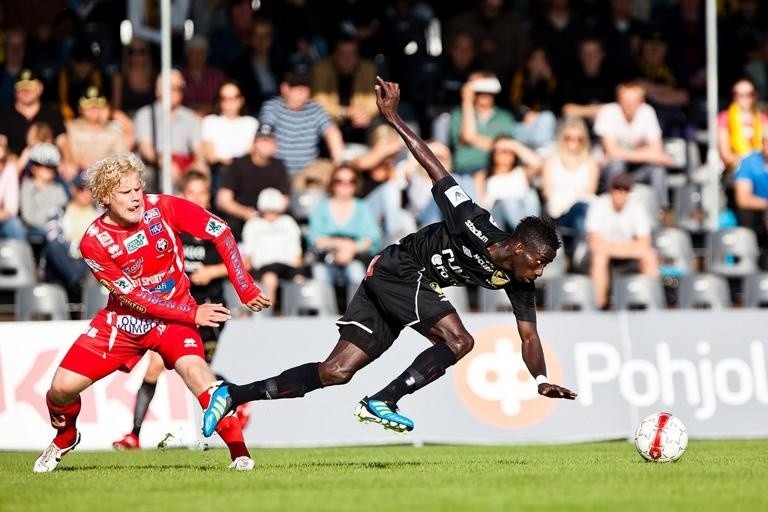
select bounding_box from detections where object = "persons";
[202,77,578,432]
[112,168,254,448]
[35,152,272,471]
[0,0,768,318]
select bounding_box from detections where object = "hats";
[78,85,108,109]
[13,68,42,93]
[253,122,281,141]
[257,185,290,215]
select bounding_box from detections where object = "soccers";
[635,412,688,463]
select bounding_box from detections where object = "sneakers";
[354,396,415,434]
[235,402,251,429]
[200,380,238,438]
[32,430,81,473]
[233,456,255,471]
[113,433,141,451]
